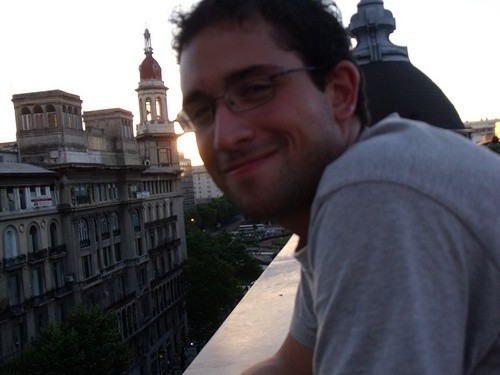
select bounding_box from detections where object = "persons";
[489,135,500,156]
[177,0,500,375]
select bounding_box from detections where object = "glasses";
[175,65,315,133]
